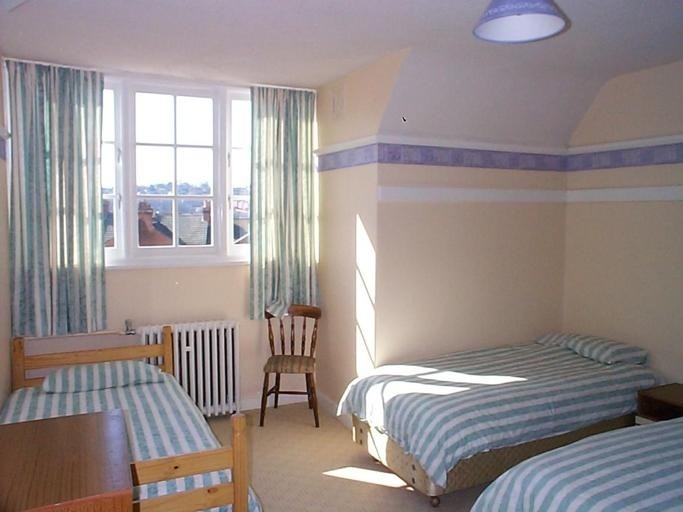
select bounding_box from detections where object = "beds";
[0,323,265,511]
[335,339,667,510]
[463,413,682,512]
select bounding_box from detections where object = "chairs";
[255,300,325,432]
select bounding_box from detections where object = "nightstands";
[630,378,683,429]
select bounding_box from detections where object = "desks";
[0,407,138,512]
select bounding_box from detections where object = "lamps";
[470,0,572,48]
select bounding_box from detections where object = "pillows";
[527,325,649,368]
[35,358,166,394]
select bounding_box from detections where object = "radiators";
[122,319,242,422]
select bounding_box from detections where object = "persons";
[197,200,247,245]
